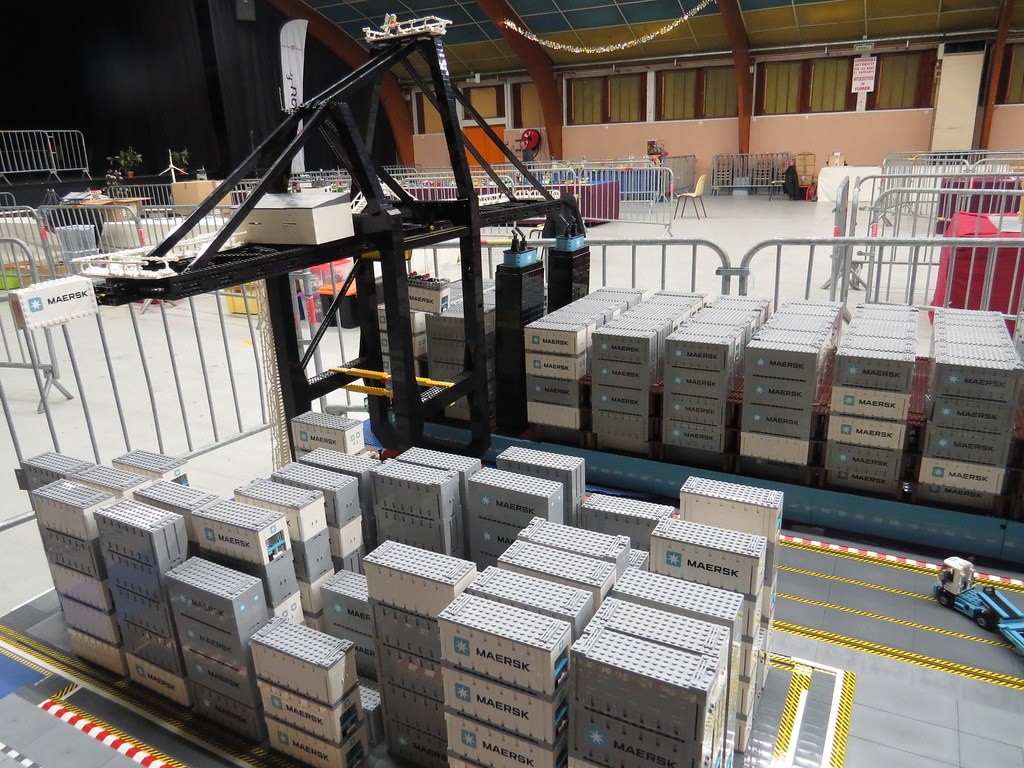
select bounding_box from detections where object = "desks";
[403,180,619,227]
[928,212,1024,336]
[936,176,1022,234]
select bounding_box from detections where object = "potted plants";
[171,149,191,175]
[108,146,143,179]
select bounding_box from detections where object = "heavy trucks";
[932,557,1024,653]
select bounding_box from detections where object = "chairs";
[768,161,795,201]
[673,174,707,219]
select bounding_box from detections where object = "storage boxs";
[229,191,355,246]
[171,182,233,215]
[0,190,154,290]
[795,152,816,185]
[227,259,386,329]
